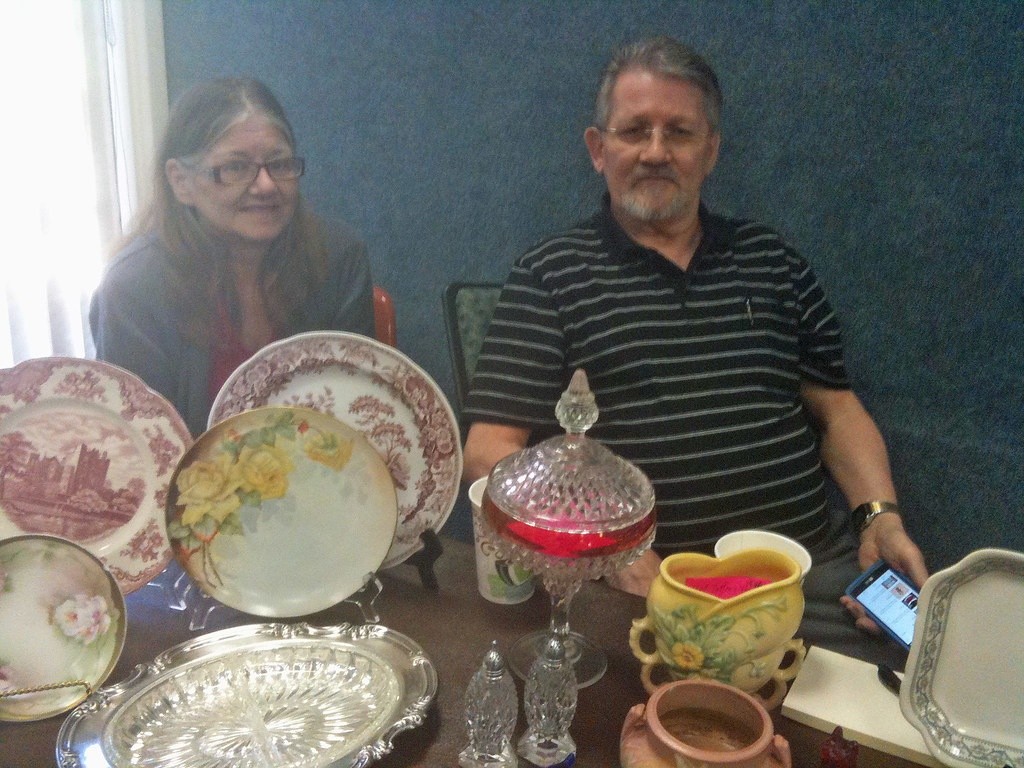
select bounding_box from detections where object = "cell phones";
[845,559,920,651]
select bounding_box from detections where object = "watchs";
[851,501,900,533]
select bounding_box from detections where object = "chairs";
[441,281,504,409]
[372,286,396,351]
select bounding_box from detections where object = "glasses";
[187,155,306,186]
[595,118,715,146]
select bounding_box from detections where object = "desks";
[0,535,924,768]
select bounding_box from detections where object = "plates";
[205,330,463,571]
[0,356,196,597]
[1,535,127,724]
[55,621,438,768]
[165,404,398,619]
[899,548,1024,768]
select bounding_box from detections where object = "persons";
[461,35,930,674]
[89,77,376,438]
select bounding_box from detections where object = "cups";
[628,547,807,713]
[468,476,536,605]
[714,529,812,577]
